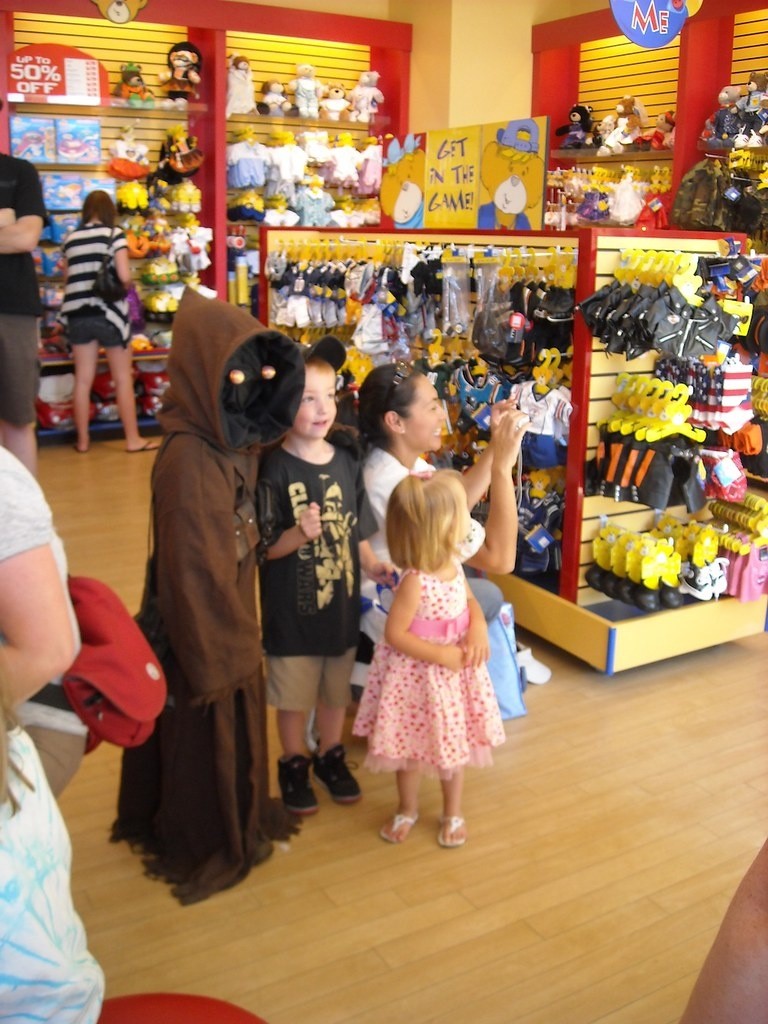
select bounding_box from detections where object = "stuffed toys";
[158,41,203,111]
[112,63,156,110]
[226,54,385,124]
[703,71,768,148]
[555,94,676,157]
[108,286,306,906]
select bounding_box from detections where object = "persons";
[61,190,160,453]
[254,335,398,815]
[358,363,532,721]
[0,101,50,479]
[678,844,768,1024]
[352,468,505,848]
[0,448,108,1024]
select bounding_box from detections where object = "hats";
[516,641,551,685]
[295,335,346,372]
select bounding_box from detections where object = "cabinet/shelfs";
[0,90,768,676]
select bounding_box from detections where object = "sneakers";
[312,744,362,804]
[278,755,317,815]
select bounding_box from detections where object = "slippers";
[437,811,466,846]
[381,814,417,841]
[127,440,162,452]
[74,440,88,452]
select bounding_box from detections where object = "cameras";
[516,414,529,428]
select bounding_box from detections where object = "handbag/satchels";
[471,577,527,719]
[63,569,167,754]
[93,267,127,303]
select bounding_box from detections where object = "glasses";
[385,359,413,411]
